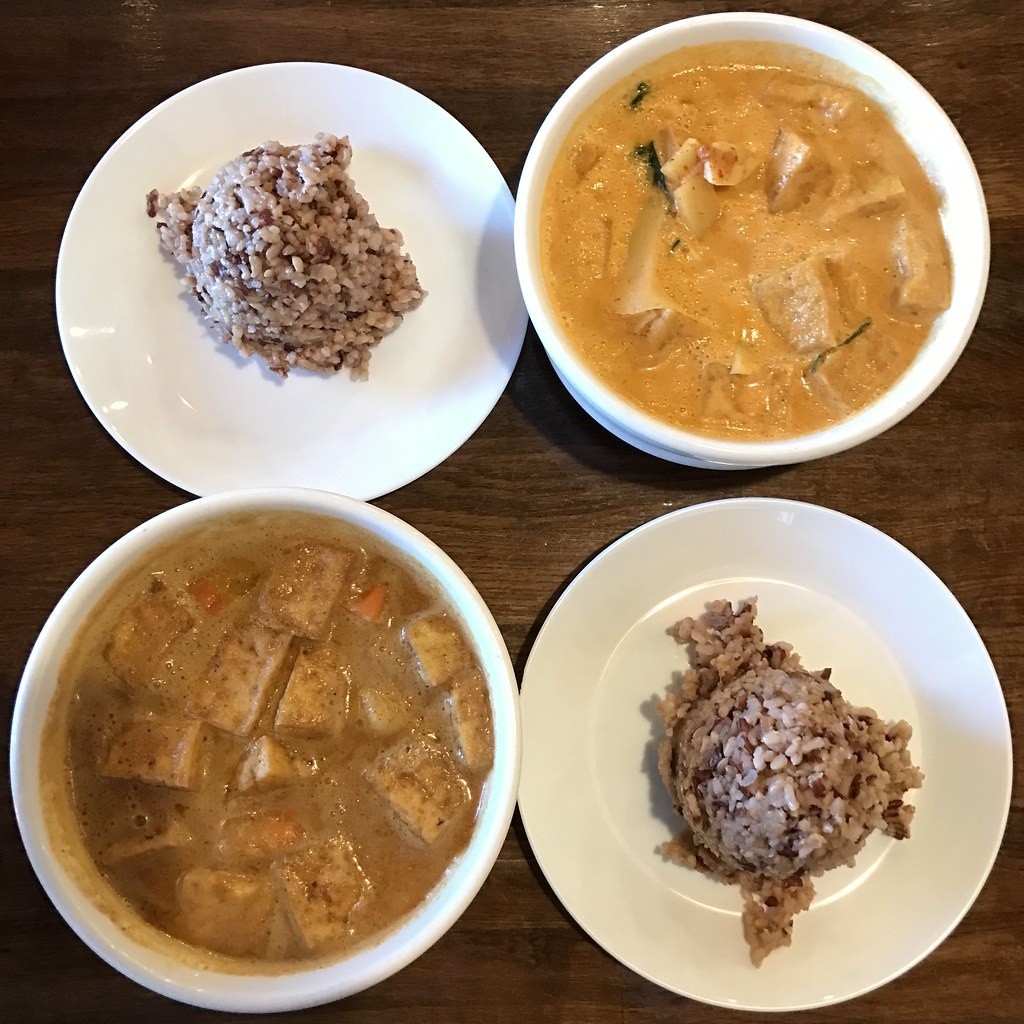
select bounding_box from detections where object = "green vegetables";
[627,82,674,207]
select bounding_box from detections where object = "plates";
[55,62,528,503]
[517,497,1014,1014]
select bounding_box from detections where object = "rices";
[145,133,430,382]
[656,596,926,965]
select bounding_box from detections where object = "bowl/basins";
[10,485,523,1015]
[515,12,991,471]
